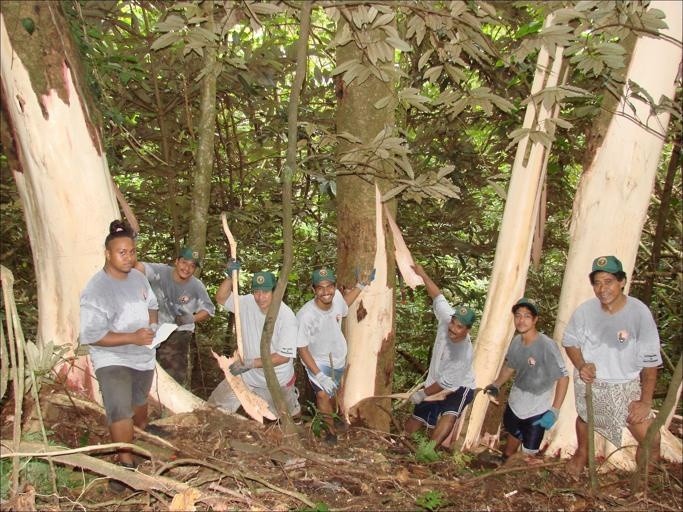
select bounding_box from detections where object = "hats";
[178,246,203,271]
[588,255,623,277]
[311,268,338,288]
[249,270,276,293]
[450,304,477,330]
[511,298,539,317]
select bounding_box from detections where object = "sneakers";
[107,457,136,496]
[325,430,338,445]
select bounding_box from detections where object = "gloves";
[175,309,193,327]
[532,407,559,430]
[408,390,428,406]
[314,372,340,398]
[226,257,240,278]
[484,380,500,397]
[229,358,255,376]
[354,268,376,289]
[150,322,161,348]
[144,264,155,282]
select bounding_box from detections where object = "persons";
[75,217,161,495]
[559,254,666,474]
[203,255,303,426]
[291,265,375,425]
[131,248,217,390]
[406,253,476,451]
[481,296,569,461]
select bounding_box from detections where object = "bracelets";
[354,282,364,292]
